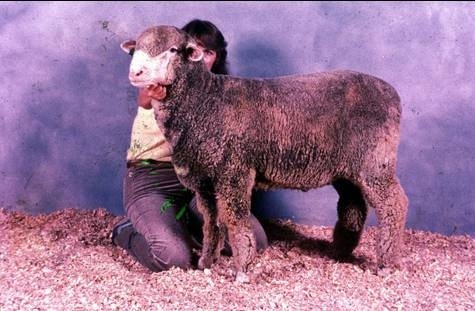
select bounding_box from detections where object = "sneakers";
[93,217,132,253]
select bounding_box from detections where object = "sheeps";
[118,23,412,285]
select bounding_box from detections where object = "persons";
[111,18,268,273]
